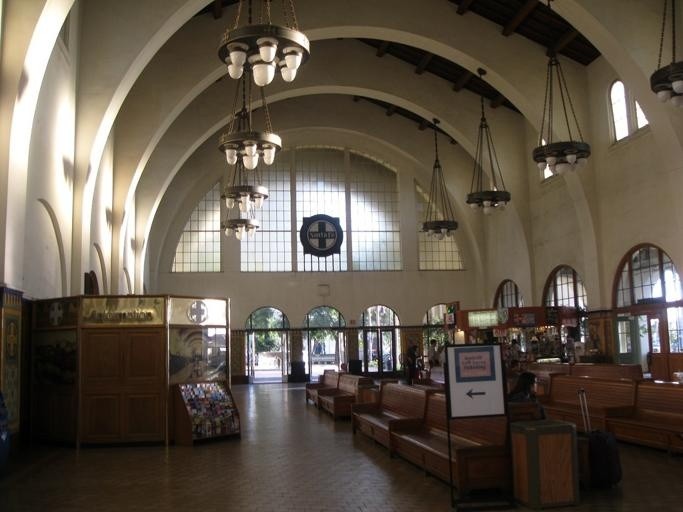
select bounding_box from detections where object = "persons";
[510,339,523,370]
[428,339,441,370]
[506,359,523,386]
[407,346,422,384]
[566,335,578,364]
[505,370,549,420]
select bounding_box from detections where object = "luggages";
[575,388,624,503]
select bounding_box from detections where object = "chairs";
[305,369,345,408]
[317,374,376,421]
[605,384,683,454]
[350,383,429,456]
[570,363,654,382]
[525,362,570,375]
[389,393,544,502]
[541,374,637,434]
[646,352,683,385]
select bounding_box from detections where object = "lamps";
[649,0,683,107]
[220,70,269,213]
[532,0,591,176]
[215,0,282,170]
[466,68,511,216]
[217,0,312,87]
[220,157,259,241]
[423,118,458,240]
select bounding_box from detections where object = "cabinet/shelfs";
[172,380,242,447]
